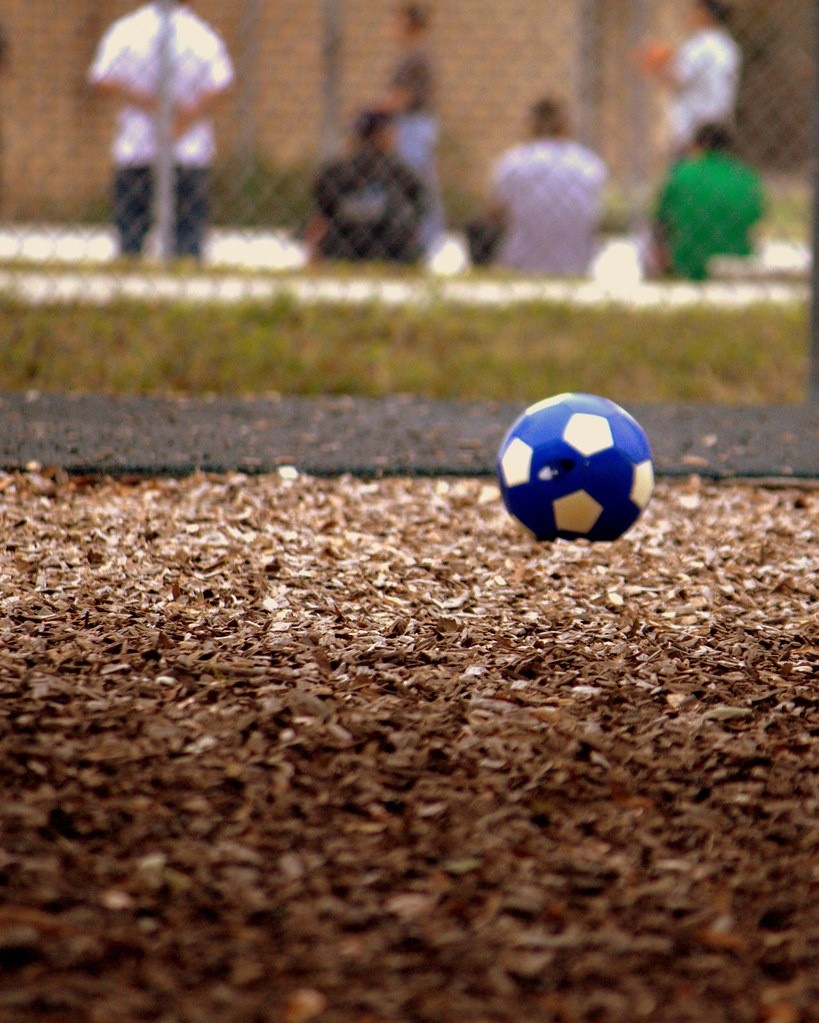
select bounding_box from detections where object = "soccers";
[496,391,657,546]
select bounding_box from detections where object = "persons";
[637,0,744,281]
[360,0,447,266]
[84,0,239,265]
[644,119,766,281]
[303,106,434,271]
[460,97,610,280]
